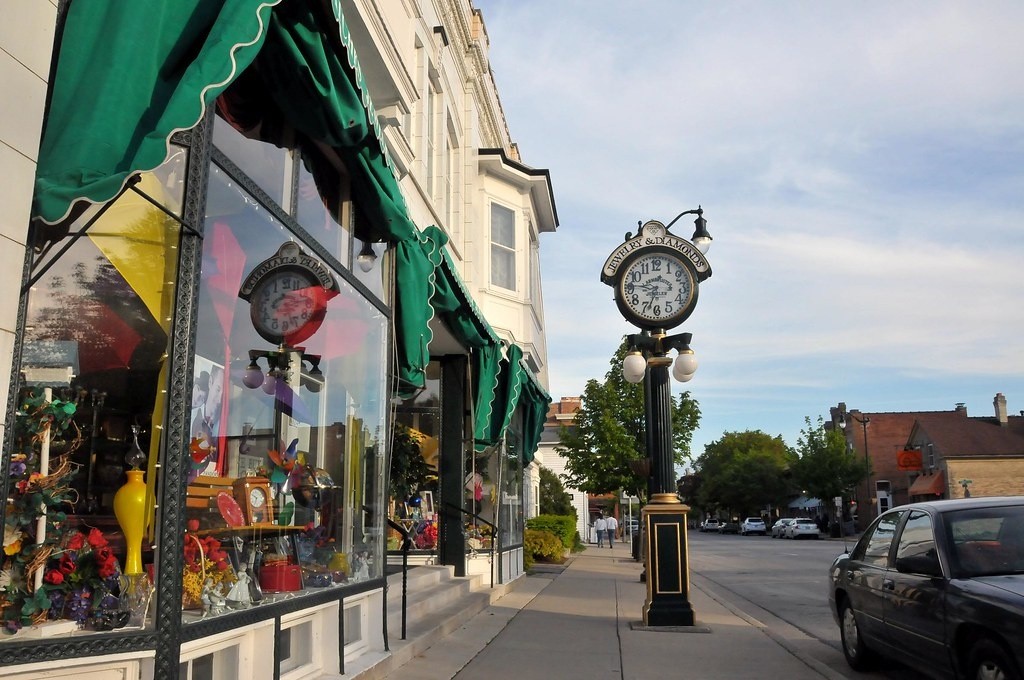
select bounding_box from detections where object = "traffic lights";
[850,500,857,506]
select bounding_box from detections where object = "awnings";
[908,470,945,495]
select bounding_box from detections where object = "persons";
[595,514,607,547]
[605,513,618,548]
[226,562,253,601]
[201,577,226,617]
[352,551,374,577]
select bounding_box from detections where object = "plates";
[217,492,245,528]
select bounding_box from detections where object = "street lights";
[640,204,715,583]
[839,410,871,525]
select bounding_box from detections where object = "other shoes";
[610,545,612,548]
[598,543,600,547]
[601,545,603,548]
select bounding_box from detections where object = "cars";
[626,520,639,534]
[771,518,793,539]
[785,518,820,540]
[828,495,1024,679]
[719,521,738,535]
[741,517,766,536]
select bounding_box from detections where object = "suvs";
[703,519,720,532]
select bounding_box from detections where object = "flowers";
[414,521,437,549]
[184,518,227,573]
[21,527,120,617]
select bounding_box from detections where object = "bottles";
[125,426,146,468]
[113,471,156,598]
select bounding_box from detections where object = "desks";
[198,525,307,590]
[398,518,430,549]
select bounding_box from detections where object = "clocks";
[232,475,274,526]
[600,219,712,330]
[237,241,340,346]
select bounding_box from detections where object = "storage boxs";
[260,565,301,592]
